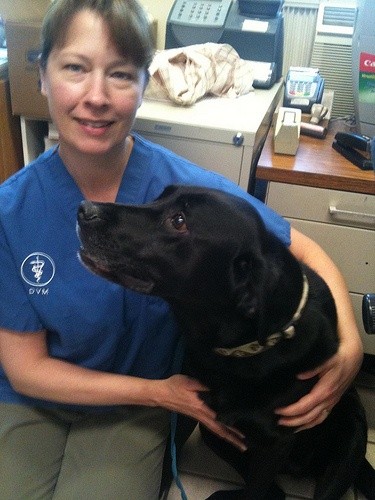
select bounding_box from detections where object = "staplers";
[332,130,375,171]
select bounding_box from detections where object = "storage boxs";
[0,0,158,184]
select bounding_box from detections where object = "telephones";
[283,64,326,110]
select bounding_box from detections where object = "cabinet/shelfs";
[256,122,375,355]
[20,51,285,192]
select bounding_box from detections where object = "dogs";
[76,185,375,500]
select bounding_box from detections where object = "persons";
[0,0,363,500]
